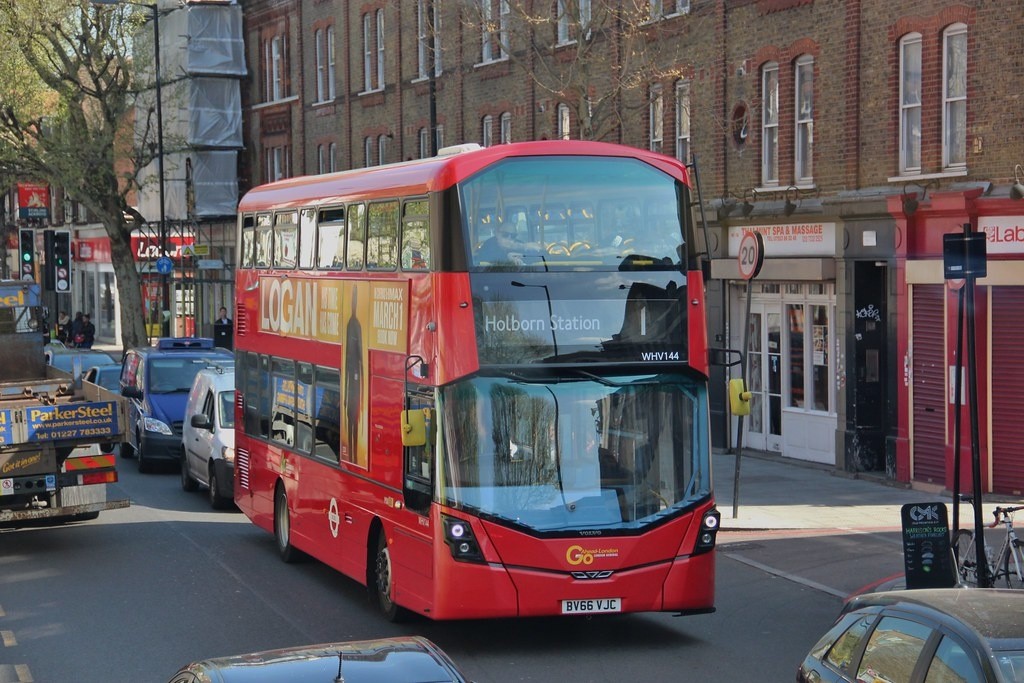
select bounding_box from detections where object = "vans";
[118,338,233,473]
[181,366,235,507]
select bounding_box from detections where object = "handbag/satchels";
[75,336,85,343]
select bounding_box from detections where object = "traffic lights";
[19,228,35,281]
[53,230,71,292]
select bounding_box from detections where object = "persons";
[344,282,365,465]
[214,307,233,352]
[41,301,95,350]
[472,220,546,266]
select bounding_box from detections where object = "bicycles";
[950,494,1024,588]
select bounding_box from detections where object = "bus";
[233,140,721,621]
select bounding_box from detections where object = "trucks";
[0,280,131,535]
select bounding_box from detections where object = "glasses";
[499,231,518,239]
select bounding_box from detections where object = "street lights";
[90,0,170,337]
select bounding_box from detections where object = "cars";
[84,363,122,395]
[44,348,118,379]
[796,588,1024,683]
[169,635,476,683]
